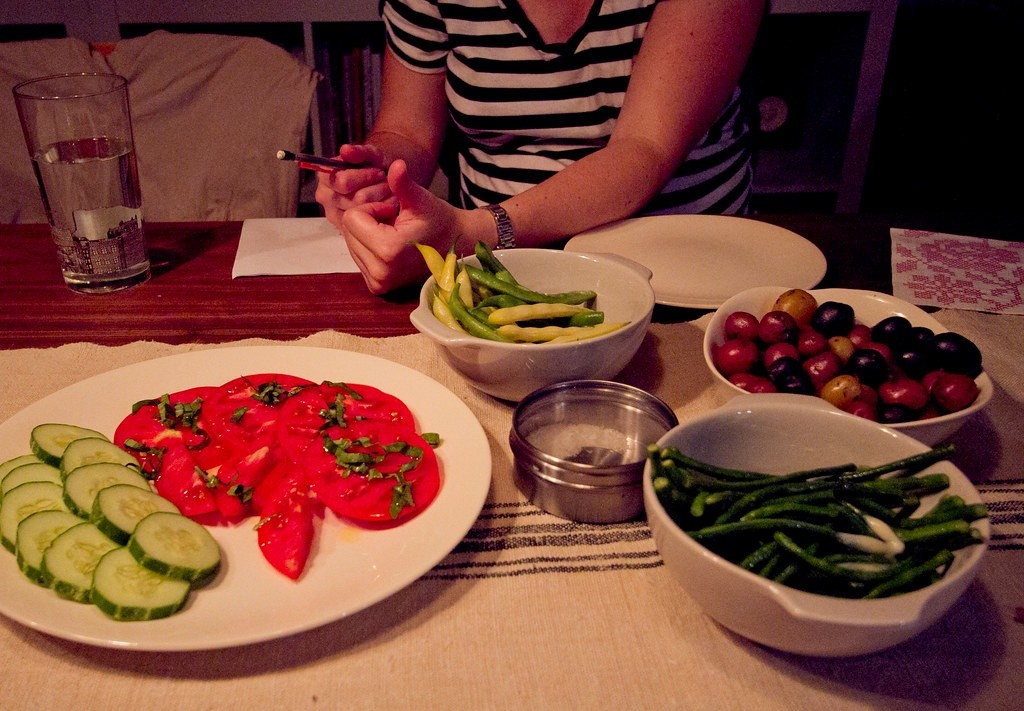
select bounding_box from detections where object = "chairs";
[0,31,323,213]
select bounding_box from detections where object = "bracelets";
[474,200,518,250]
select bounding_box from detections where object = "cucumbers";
[1,422,220,623]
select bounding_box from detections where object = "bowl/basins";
[701,286,991,446]
[508,379,680,523]
[408,248,656,403]
[643,395,989,658]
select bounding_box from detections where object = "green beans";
[405,234,634,344]
[648,442,987,599]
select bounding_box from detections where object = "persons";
[314,1,773,295]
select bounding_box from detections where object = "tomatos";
[716,291,984,423]
[113,373,442,581]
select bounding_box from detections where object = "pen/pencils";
[276,150,391,175]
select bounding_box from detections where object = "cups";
[12,71,152,294]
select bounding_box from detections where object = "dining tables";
[2,218,1024,711]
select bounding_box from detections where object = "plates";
[562,214,828,309]
[1,346,496,650]
